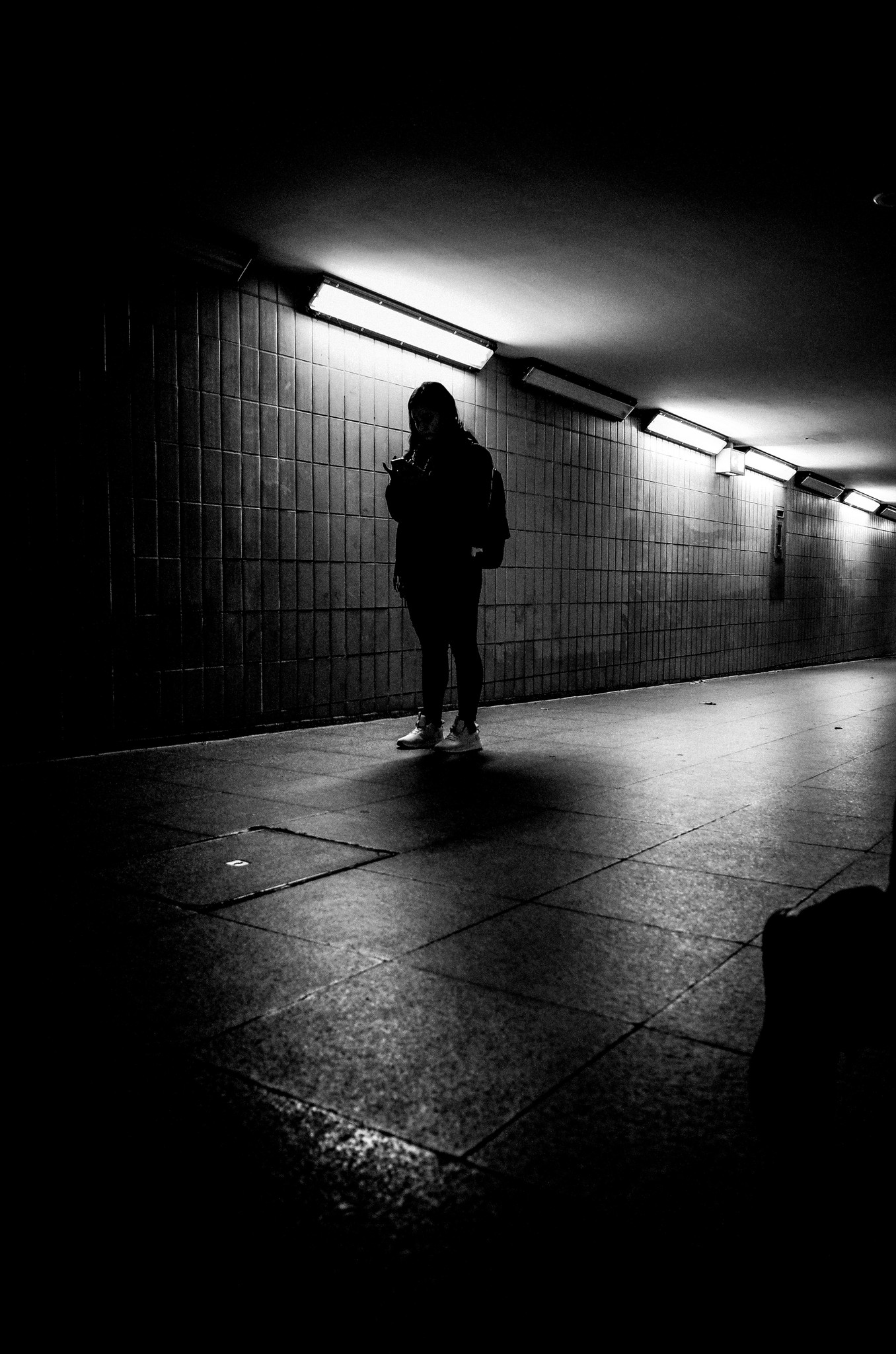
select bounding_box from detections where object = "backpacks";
[478,469,511,569]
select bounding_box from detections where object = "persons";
[383,381,494,752]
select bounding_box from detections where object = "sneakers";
[433,716,483,752]
[397,711,445,748]
